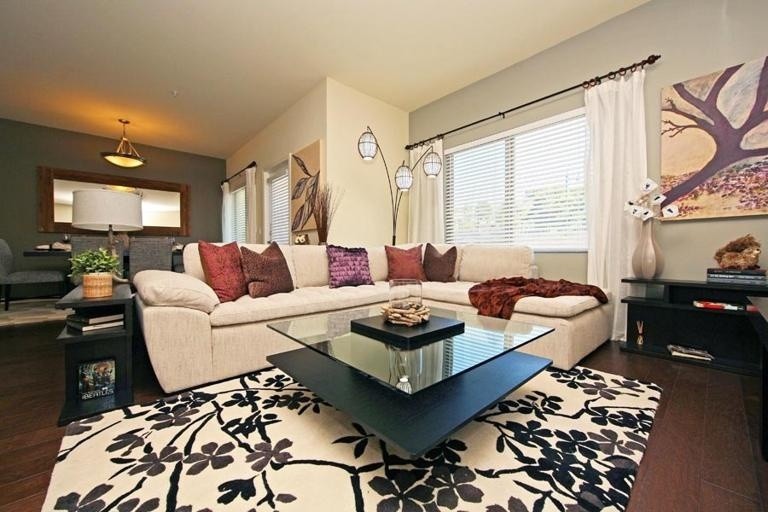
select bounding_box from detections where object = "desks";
[23,246,183,271]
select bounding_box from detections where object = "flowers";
[624,178,679,221]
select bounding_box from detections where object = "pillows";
[423,243,457,282]
[325,243,375,288]
[241,242,294,298]
[197,240,248,303]
[385,245,426,284]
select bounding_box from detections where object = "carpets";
[40,366,663,512]
[0,299,75,326]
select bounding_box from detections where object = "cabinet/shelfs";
[55,283,134,427]
[621,278,768,377]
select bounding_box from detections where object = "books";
[693,300,758,312]
[67,312,125,324]
[65,320,125,332]
[706,267,768,288]
[666,342,714,361]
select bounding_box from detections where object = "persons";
[81,364,112,394]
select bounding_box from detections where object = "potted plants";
[66,247,123,299]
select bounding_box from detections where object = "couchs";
[134,243,611,394]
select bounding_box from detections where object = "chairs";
[130,237,173,282]
[0,239,65,310]
[71,238,124,283]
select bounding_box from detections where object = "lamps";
[358,127,442,246]
[74,191,143,285]
[101,119,145,168]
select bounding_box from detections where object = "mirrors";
[36,166,190,237]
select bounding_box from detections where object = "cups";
[388,279,423,310]
[63,234,72,243]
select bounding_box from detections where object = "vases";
[631,218,665,279]
